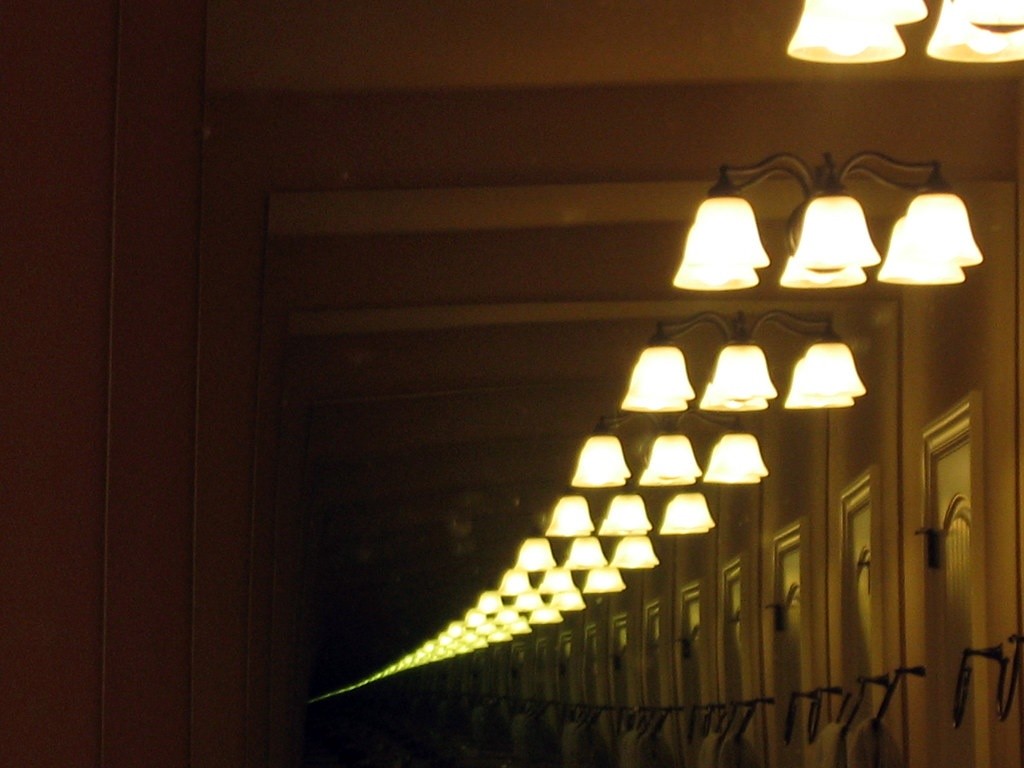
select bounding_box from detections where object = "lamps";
[787,0,1024,65]
[449,309,872,650]
[672,146,983,291]
